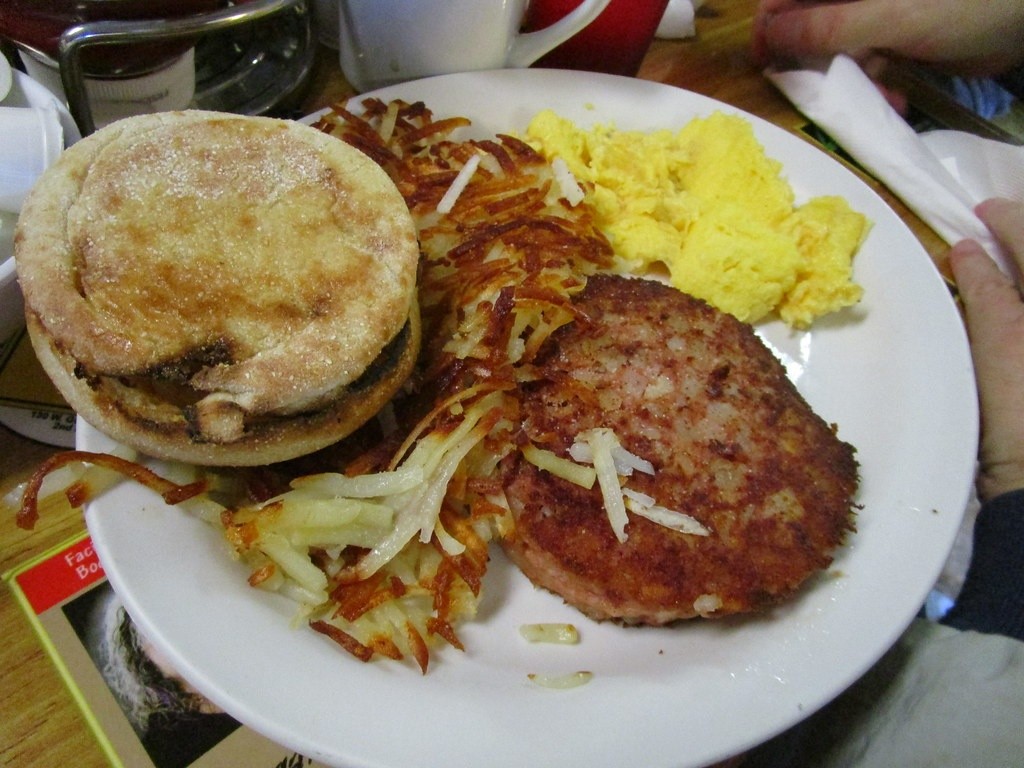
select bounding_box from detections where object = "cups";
[338,0,609,95]
[526,0,670,78]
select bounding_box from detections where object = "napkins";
[764,53,1024,283]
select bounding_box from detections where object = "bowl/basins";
[0,70,83,331]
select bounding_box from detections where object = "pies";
[11,107,420,463]
[15,95,622,675]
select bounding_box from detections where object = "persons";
[749,1,1024,124]
[817,197,1024,768]
[102,598,244,768]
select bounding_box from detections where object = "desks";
[0,0,951,768]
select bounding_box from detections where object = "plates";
[76,67,978,768]
